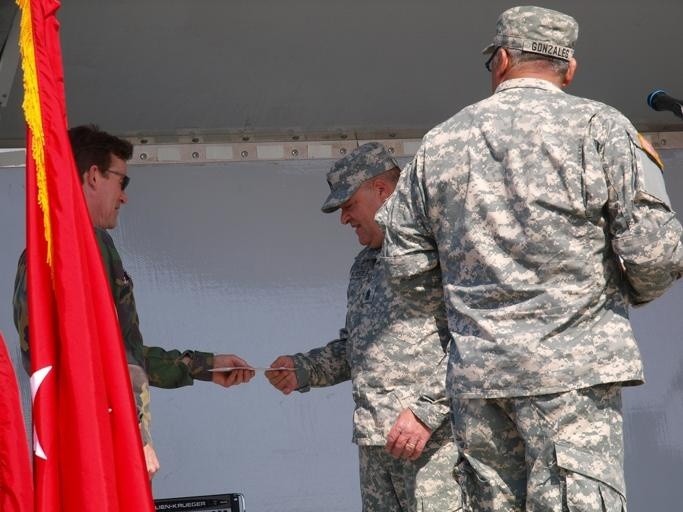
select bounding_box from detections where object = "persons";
[265,142,467,511]
[375,6,683,510]
[14,122,253,492]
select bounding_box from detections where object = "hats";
[321,142,399,213]
[482,6,579,61]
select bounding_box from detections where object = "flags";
[15,0,158,511]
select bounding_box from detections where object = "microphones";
[646,88,683,112]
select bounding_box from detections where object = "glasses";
[105,169,130,189]
[484,46,503,72]
